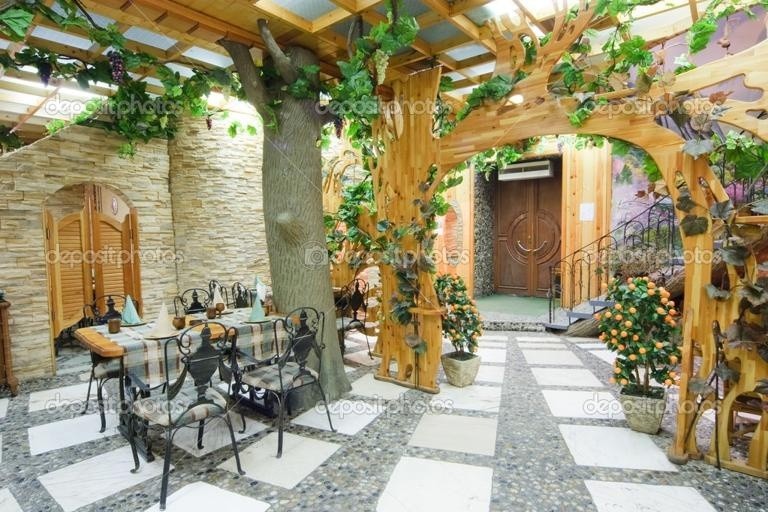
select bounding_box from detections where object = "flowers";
[594,274,685,391]
[432,273,483,355]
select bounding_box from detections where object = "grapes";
[334,116,344,138]
[206,119,212,129]
[39,63,52,86]
[109,53,125,83]
[373,49,389,86]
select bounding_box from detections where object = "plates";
[115,319,148,327]
[242,315,273,323]
[144,330,180,339]
[204,309,234,315]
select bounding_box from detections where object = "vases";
[439,350,480,385]
[620,383,665,435]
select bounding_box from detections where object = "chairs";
[73,278,370,510]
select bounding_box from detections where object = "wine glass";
[215,302,225,319]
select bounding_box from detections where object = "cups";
[173,317,185,330]
[208,307,216,319]
[108,318,121,333]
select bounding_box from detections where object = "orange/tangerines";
[443,275,482,353]
[593,276,678,386]
